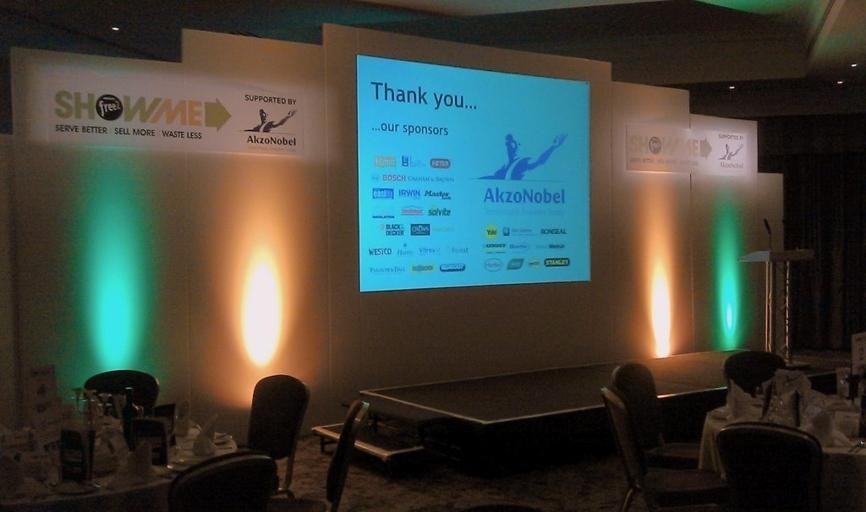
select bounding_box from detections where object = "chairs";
[602,350,822,509]
[166,449,278,510]
[264,399,371,510]
[81,370,161,416]
[236,374,310,490]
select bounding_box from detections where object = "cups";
[58,398,97,485]
[195,438,216,455]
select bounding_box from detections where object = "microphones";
[761,218,776,252]
[781,218,796,250]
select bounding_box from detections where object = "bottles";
[72,387,138,452]
